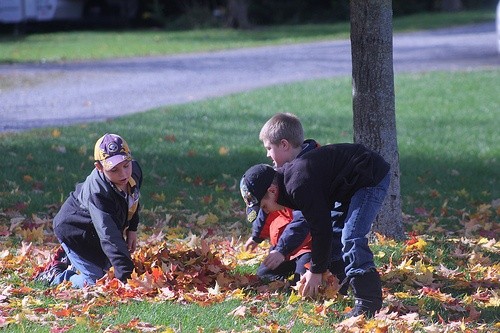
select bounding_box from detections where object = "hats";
[240,164,274,222]
[93,132,132,171]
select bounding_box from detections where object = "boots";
[338,270,383,319]
[329,259,348,296]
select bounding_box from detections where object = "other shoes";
[39,260,66,285]
[45,246,69,270]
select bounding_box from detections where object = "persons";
[243,114,321,281]
[34,134,143,289]
[240,144,390,319]
